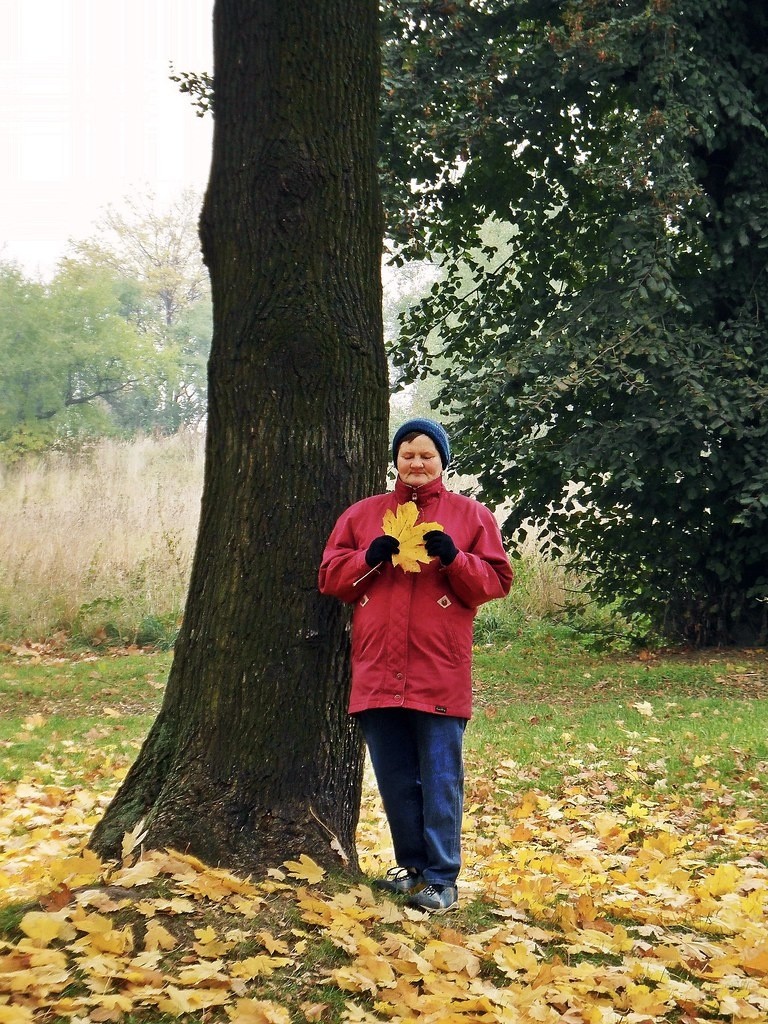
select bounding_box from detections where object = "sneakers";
[371,866,425,896]
[407,883,460,917]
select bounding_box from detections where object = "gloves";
[423,529,459,566]
[365,535,400,571]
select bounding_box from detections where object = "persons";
[319,418,513,914]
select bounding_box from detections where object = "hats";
[392,417,451,471]
